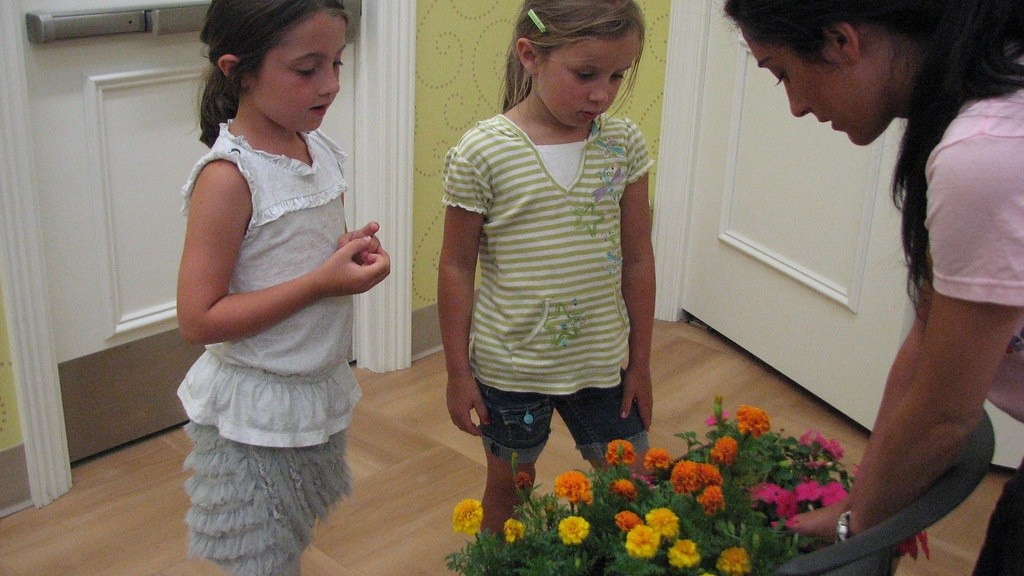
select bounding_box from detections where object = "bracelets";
[835,510,848,545]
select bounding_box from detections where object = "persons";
[718,0,1024,575]
[438,0,659,555]
[170,0,396,576]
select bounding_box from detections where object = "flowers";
[445,389,931,576]
